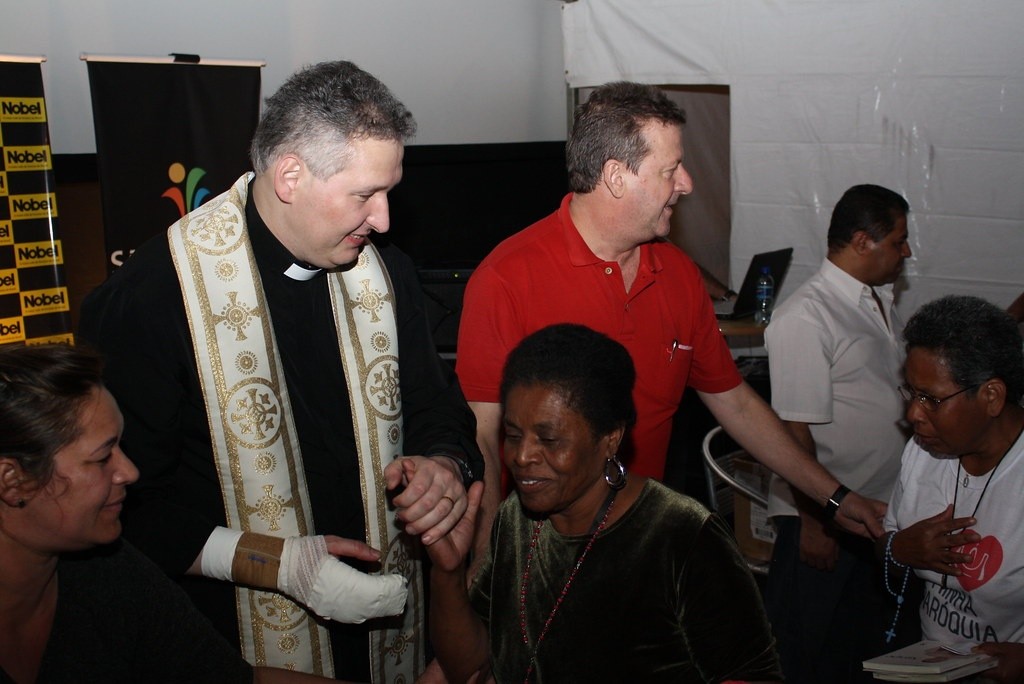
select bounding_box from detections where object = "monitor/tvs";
[389,140,568,283]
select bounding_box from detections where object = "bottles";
[754,266,774,326]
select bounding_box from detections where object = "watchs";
[427,448,474,489]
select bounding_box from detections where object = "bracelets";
[721,290,737,302]
[884,530,911,642]
[826,486,851,514]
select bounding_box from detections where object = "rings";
[443,497,455,507]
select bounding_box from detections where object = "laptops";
[712,247,795,320]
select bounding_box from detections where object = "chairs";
[702,425,794,575]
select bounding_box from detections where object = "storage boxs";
[732,456,786,561]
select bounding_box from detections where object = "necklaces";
[942,428,1023,589]
[519,491,616,684]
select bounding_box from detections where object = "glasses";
[896,384,981,412]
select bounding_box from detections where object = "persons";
[402,323,782,684]
[0,342,343,684]
[92,62,484,684]
[877,294,1024,684]
[764,184,917,684]
[456,83,888,542]
